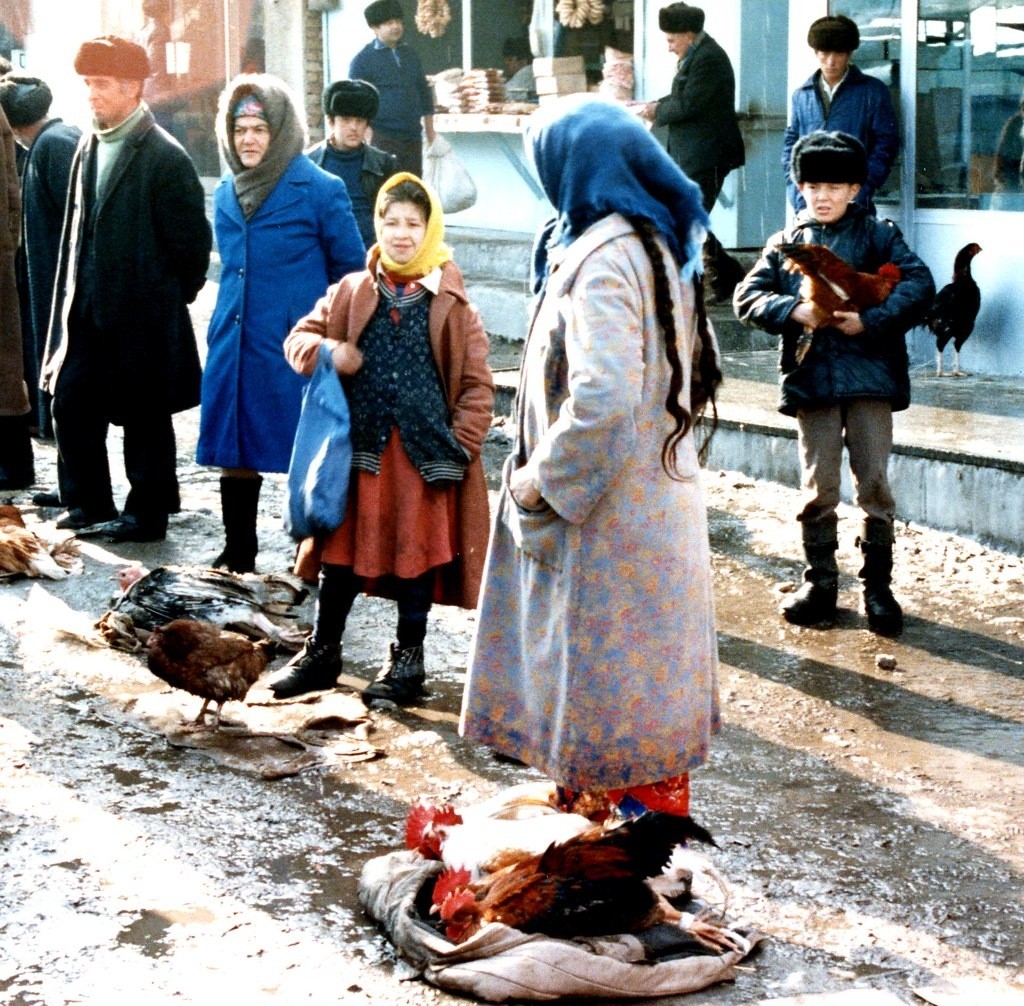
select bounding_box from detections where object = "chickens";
[774,243,903,365]
[0,496,76,582]
[430,810,750,956]
[96,564,309,655]
[143,621,277,736]
[408,805,595,875]
[914,242,982,380]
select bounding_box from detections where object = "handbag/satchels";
[285,347,351,542]
[422,134,479,214]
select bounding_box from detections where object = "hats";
[659,2,706,33]
[232,90,268,123]
[0,75,53,126]
[364,0,402,28]
[323,79,381,120]
[75,34,150,80]
[790,128,868,186]
[808,14,860,51]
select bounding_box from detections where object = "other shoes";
[704,290,731,306]
[34,488,68,508]
[57,502,120,529]
[105,511,170,542]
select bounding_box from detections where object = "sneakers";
[266,640,344,697]
[363,641,429,699]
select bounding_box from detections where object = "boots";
[212,474,264,570]
[780,537,838,617]
[855,535,905,632]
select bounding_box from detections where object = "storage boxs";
[538,95,563,107]
[532,55,585,76]
[534,74,588,95]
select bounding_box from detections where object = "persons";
[348,0,435,181]
[458,99,726,832]
[501,37,537,100]
[733,128,937,632]
[194,73,367,577]
[264,169,494,702]
[302,79,401,253]
[780,14,880,229]
[635,0,746,304]
[0,20,213,544]
